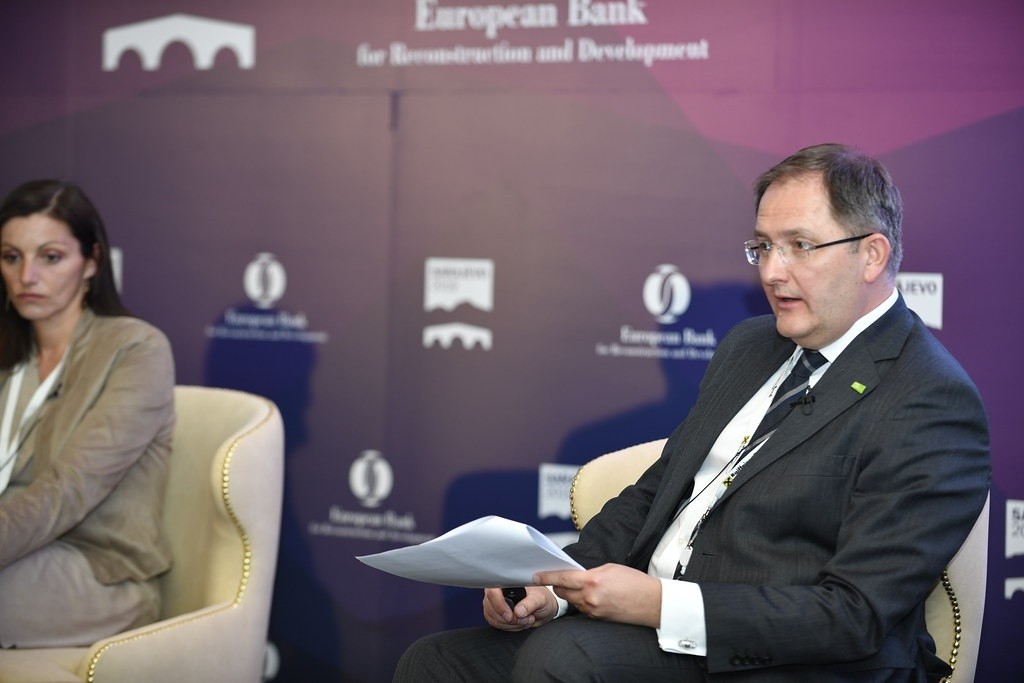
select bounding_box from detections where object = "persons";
[394,141,993,683]
[0,179,176,650]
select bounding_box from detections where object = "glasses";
[744,231,878,266]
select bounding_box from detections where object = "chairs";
[570,437,991,682]
[0,385,283,683]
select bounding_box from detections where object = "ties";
[732,348,829,468]
